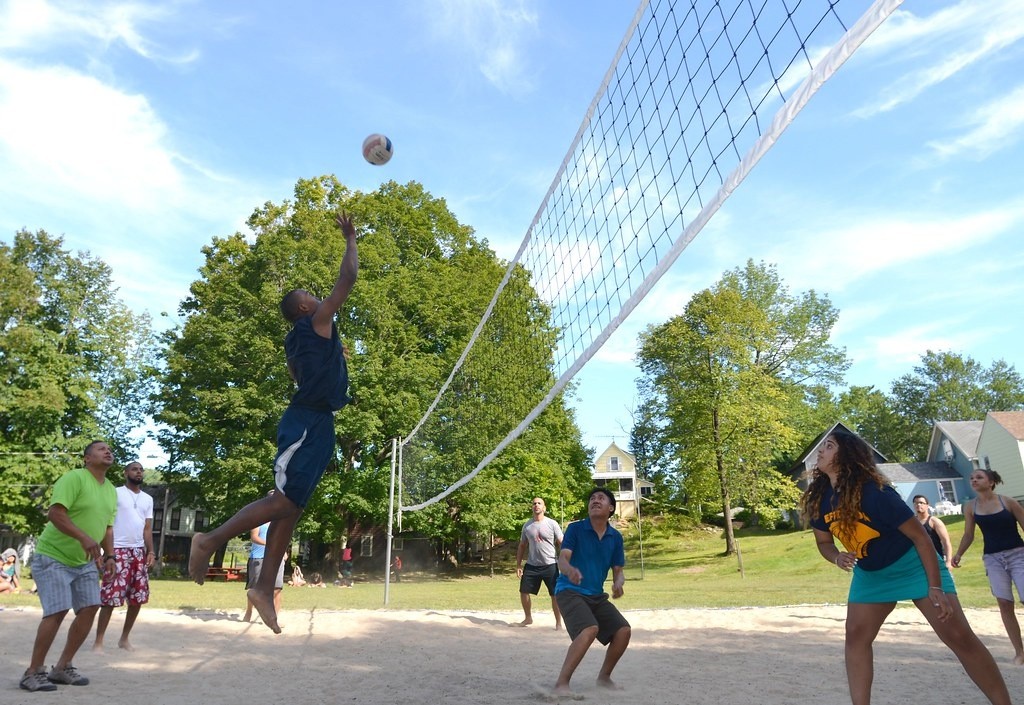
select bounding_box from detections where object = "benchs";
[206,572,228,582]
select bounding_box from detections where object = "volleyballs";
[362,133,395,165]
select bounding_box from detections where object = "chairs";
[929,501,962,516]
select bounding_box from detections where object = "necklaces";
[128,490,139,508]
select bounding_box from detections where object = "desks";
[208,567,242,571]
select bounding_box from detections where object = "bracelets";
[929,587,943,591]
[148,552,155,555]
[835,557,838,566]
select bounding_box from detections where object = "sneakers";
[19,665,57,692]
[47,663,90,685]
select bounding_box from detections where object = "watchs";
[103,556,116,563]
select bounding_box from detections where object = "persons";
[0,548,20,593]
[242,490,288,628]
[288,566,306,587]
[390,555,401,582]
[801,432,1011,705]
[19,441,117,691]
[517,497,564,630]
[911,495,953,578]
[950,469,1024,663]
[552,487,631,695]
[307,572,326,588]
[188,209,361,634]
[92,462,154,655]
